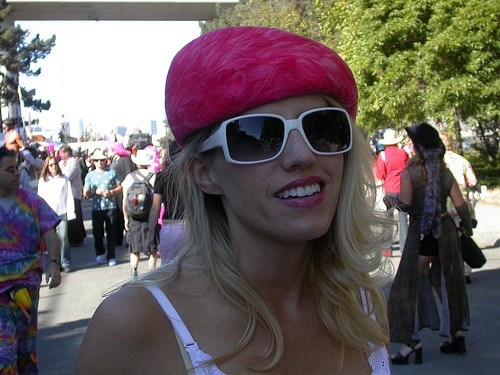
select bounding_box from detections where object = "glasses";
[2,164,19,173]
[48,163,58,166]
[94,159,107,162]
[195,107,355,167]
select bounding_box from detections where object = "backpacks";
[124,170,155,221]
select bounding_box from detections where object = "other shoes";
[64,266,71,272]
[381,249,392,257]
[131,267,139,280]
[96,255,105,264]
[108,258,116,266]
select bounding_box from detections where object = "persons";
[386,123,475,366]
[70,24,394,375]
[0,118,194,272]
[372,126,482,284]
[35,156,78,274]
[119,148,162,281]
[81,149,124,267]
[1,146,63,375]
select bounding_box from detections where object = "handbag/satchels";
[459,234,487,270]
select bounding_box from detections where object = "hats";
[166,25,357,148]
[406,122,446,160]
[130,149,154,166]
[378,128,404,147]
[91,149,108,160]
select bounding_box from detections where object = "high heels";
[390,338,423,366]
[439,335,468,355]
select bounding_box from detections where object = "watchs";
[49,257,62,267]
